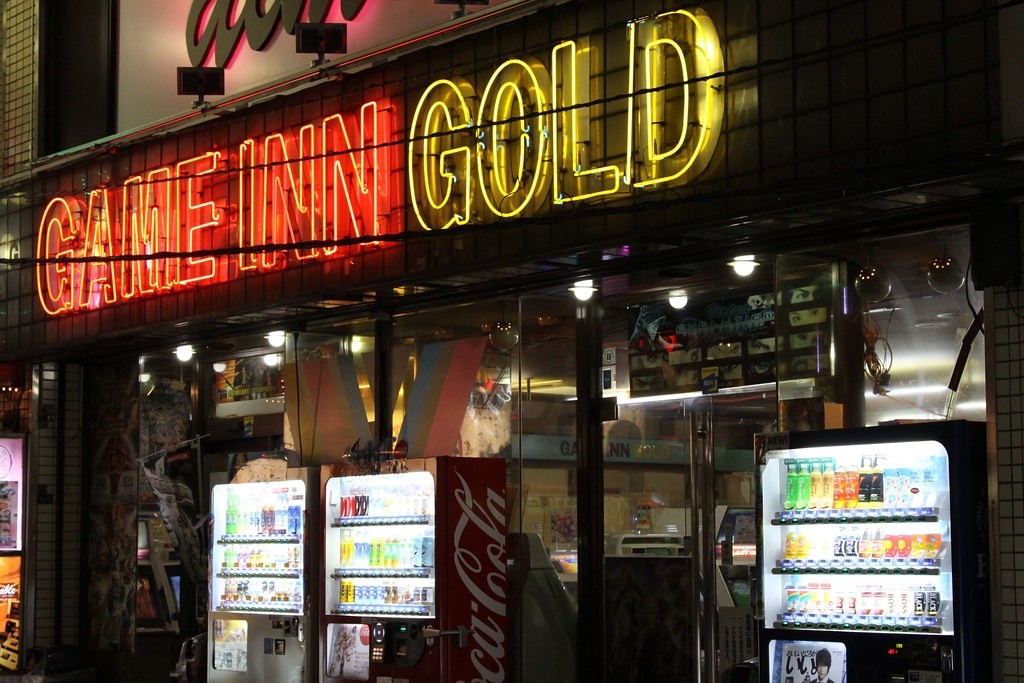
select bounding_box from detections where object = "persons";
[720,364,742,379]
[780,307,828,329]
[628,305,666,352]
[809,649,834,683]
[639,354,660,367]
[781,286,824,305]
[674,370,699,386]
[632,375,664,391]
[783,331,828,350]
[713,342,741,358]
[786,354,827,373]
[746,361,775,377]
[747,339,776,354]
[234,365,277,401]
[747,293,775,310]
[669,349,701,365]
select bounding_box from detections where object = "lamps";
[263,336,285,347]
[567,287,599,300]
[726,260,761,276]
[172,351,197,361]
[668,295,691,308]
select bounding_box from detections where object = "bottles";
[340,578,429,606]
[221,546,300,569]
[781,525,939,570]
[779,454,938,520]
[339,535,413,566]
[225,493,288,538]
[779,577,941,628]
[715,540,722,565]
[221,580,301,609]
[638,505,651,534]
[630,512,638,534]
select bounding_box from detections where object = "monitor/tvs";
[158,561,182,632]
